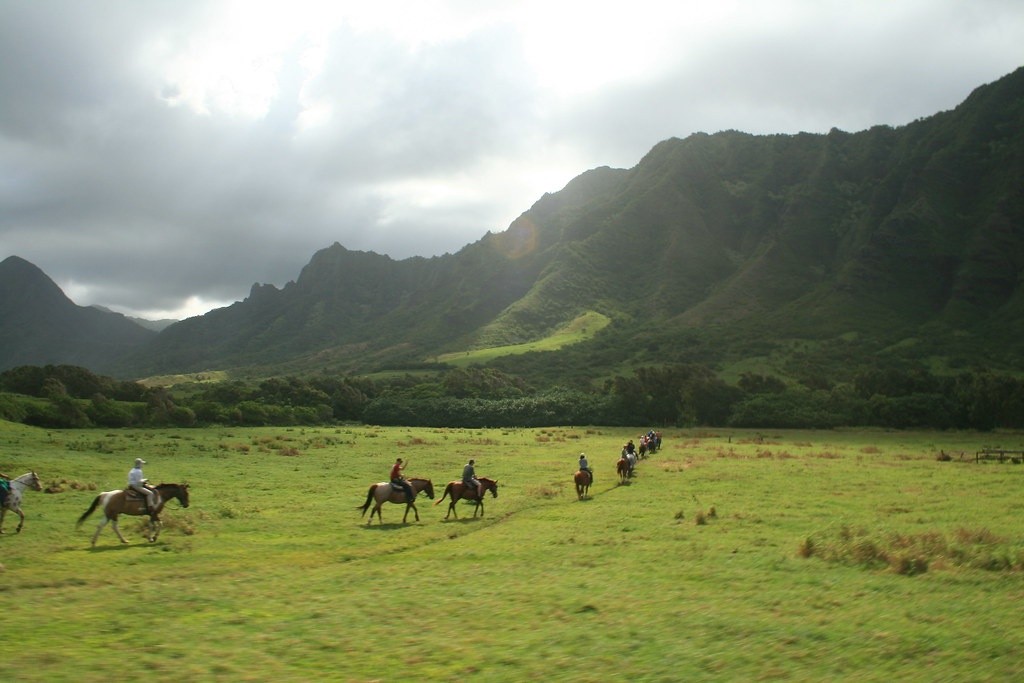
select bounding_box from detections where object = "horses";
[624,450,637,472]
[574,466,594,500]
[75,483,191,549]
[615,458,629,485]
[433,478,499,520]
[637,430,660,459]
[0,469,43,534]
[356,478,435,528]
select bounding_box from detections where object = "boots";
[148,506,154,515]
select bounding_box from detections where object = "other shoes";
[408,498,413,502]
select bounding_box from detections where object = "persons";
[390,458,414,501]
[639,429,663,455]
[0,472,12,507]
[128,457,156,516]
[579,453,593,483]
[624,440,638,463]
[621,445,632,469]
[463,460,484,499]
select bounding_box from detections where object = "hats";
[396,458,402,462]
[470,460,474,462]
[630,440,633,443]
[581,453,584,457]
[135,458,146,465]
